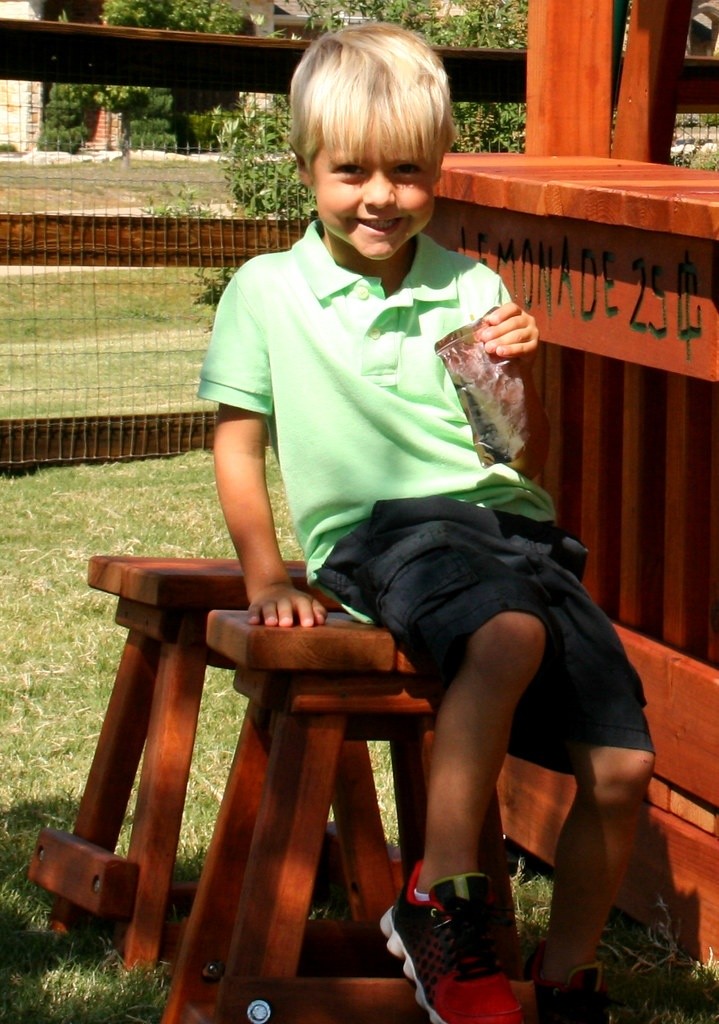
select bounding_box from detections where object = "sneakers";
[379,858,525,1024]
[523,940,610,1024]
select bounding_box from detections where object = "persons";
[209,25,656,1024]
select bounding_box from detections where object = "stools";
[26,556,538,1024]
